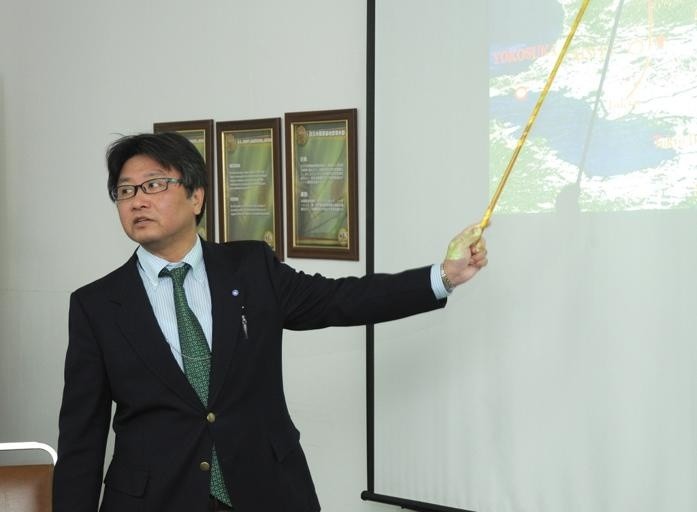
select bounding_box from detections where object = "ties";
[160,263,235,510]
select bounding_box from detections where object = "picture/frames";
[150,108,360,264]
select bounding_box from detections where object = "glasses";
[110,177,183,200]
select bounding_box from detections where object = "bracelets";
[441,264,452,288]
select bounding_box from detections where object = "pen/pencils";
[241,306,248,337]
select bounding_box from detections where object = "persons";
[52,132,487,511]
